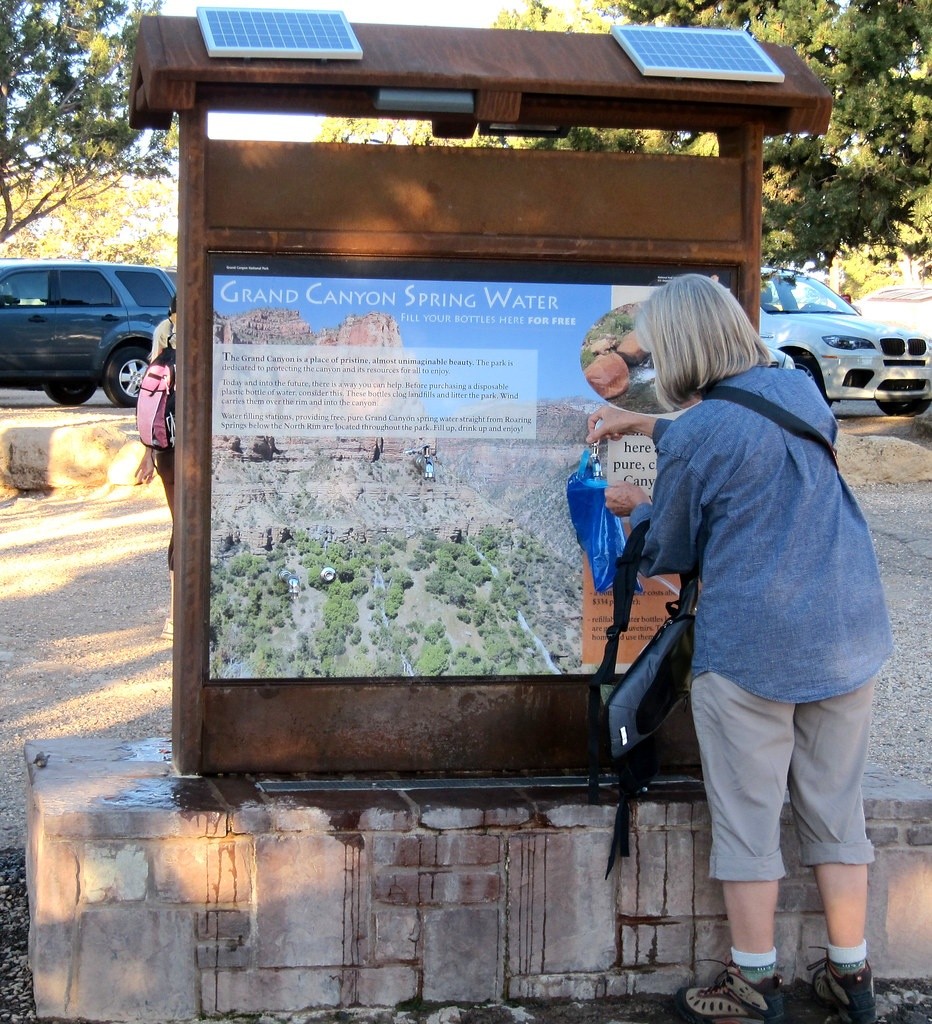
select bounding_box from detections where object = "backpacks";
[136,334,177,451]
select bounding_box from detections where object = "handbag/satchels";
[587,518,694,879]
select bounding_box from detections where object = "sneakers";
[806,944,877,1023]
[675,954,786,1022]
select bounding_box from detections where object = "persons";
[134,324,176,643]
[586,274,893,1024]
[146,291,176,365]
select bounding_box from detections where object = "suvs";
[759,267,932,417]
[0,262,177,408]
